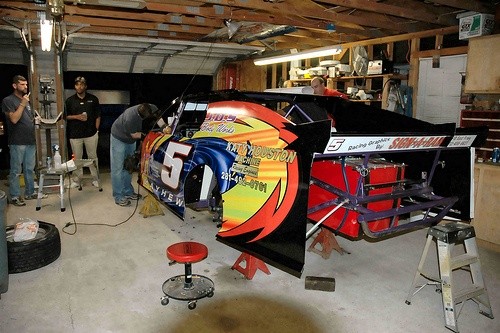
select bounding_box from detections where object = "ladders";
[405,222,494,333]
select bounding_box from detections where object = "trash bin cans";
[0,190,9,293]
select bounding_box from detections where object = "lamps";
[38,12,54,51]
[253,46,343,66]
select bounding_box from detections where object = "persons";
[110,103,171,206]
[311,77,349,127]
[63,76,103,191]
[2,75,38,206]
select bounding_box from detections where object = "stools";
[160,242,215,310]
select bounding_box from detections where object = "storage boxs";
[459,14,494,40]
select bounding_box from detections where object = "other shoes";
[115,198,131,206]
[92,181,100,188]
[125,192,142,200]
[65,182,80,189]
[8,196,26,205]
[26,192,46,199]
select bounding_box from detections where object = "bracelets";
[20,103,25,107]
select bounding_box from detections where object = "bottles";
[45,156,52,172]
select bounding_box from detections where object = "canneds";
[492,148,499,162]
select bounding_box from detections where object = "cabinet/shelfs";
[460,35,500,244]
[287,75,407,110]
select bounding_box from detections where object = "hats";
[74,76,87,84]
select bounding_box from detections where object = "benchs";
[36,159,103,212]
